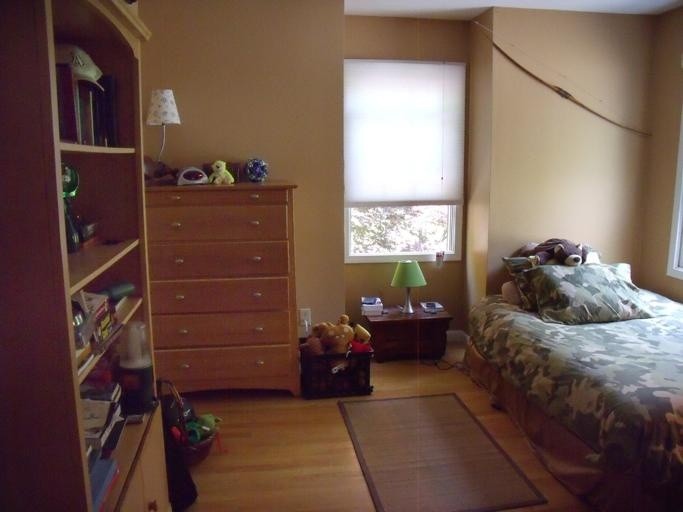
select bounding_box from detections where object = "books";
[55,62,120,148]
[76,290,128,475]
[82,222,98,248]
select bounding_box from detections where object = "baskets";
[156,378,219,466]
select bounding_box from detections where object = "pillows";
[501,248,654,326]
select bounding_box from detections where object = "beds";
[469,286,683,512]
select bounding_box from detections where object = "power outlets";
[299,307,312,327]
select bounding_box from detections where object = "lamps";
[145,90,181,180]
[391,259,427,314]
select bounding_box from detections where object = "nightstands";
[362,304,450,363]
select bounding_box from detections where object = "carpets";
[331,393,549,511]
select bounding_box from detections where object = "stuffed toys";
[544,244,586,268]
[299,314,372,395]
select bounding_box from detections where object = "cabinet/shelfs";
[1,3,180,512]
[144,179,300,399]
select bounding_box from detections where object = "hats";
[54,43,104,93]
